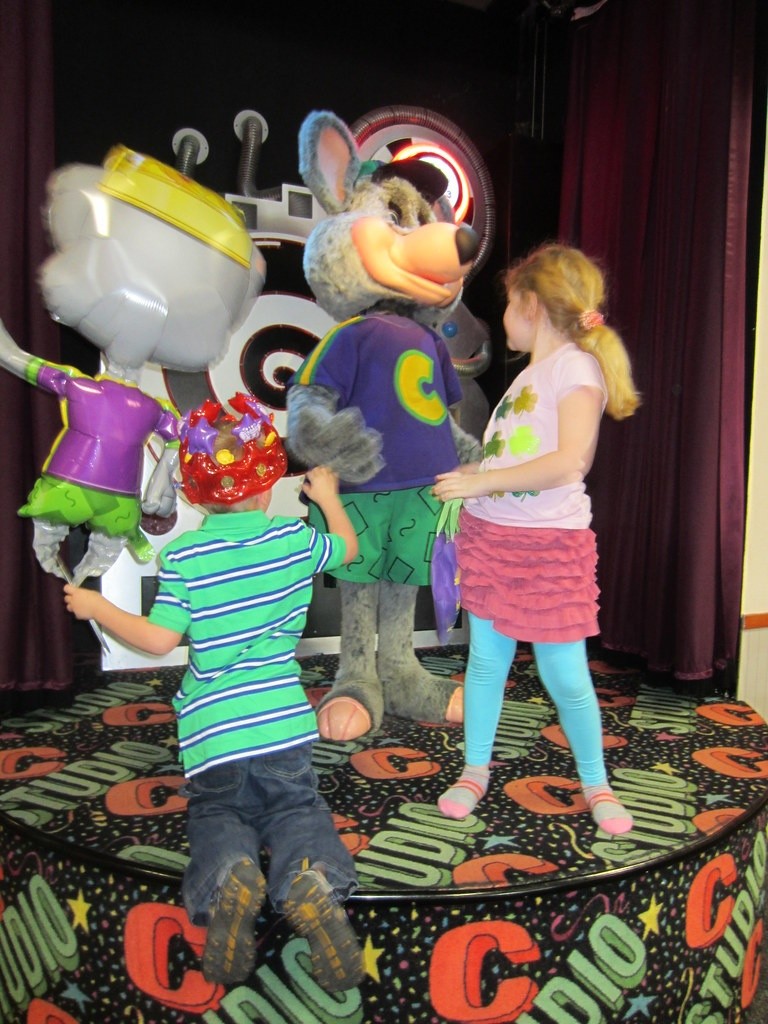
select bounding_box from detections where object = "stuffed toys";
[284,112,489,748]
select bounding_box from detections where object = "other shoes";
[204,858,266,987]
[284,868,363,993]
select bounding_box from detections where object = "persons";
[428,242,639,834]
[65,391,365,993]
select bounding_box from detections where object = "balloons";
[1,144,268,581]
